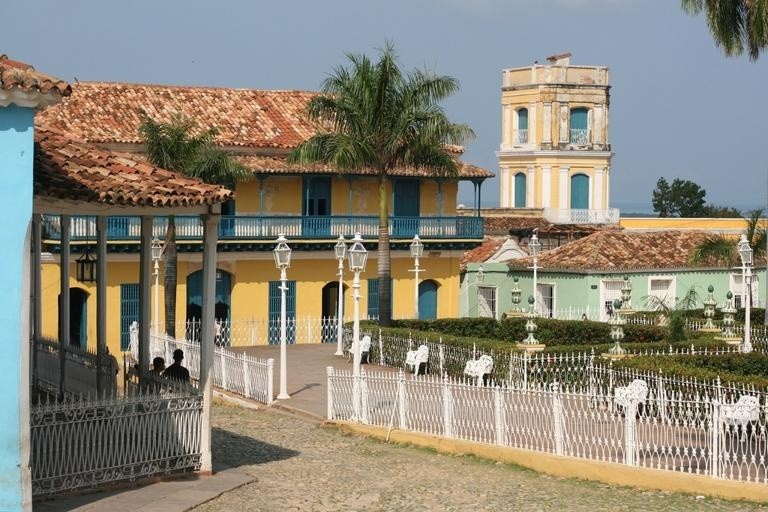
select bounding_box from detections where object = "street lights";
[334,234,349,357]
[347,231,369,424]
[528,234,543,314]
[272,233,292,399]
[151,237,162,358]
[408,234,426,319]
[738,233,753,354]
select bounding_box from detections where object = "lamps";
[76,216,97,284]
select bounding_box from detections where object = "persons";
[147,357,166,393]
[97,345,119,405]
[159,349,190,394]
[534,61,538,66]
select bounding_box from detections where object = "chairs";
[463,355,493,389]
[716,395,761,446]
[611,378,649,421]
[347,336,371,365]
[403,345,429,377]
